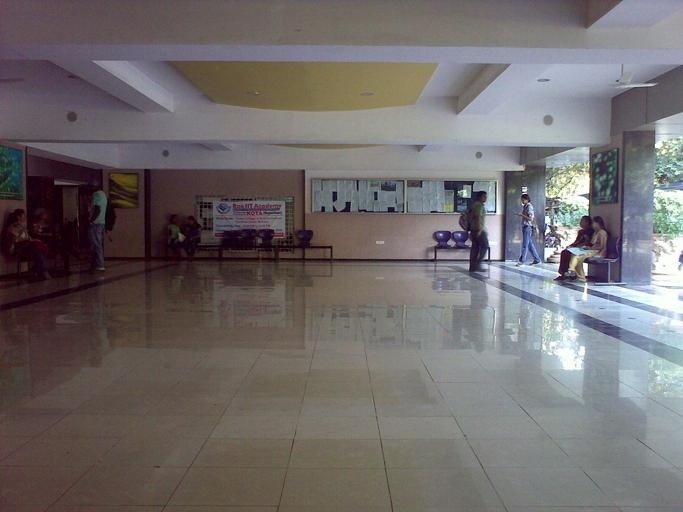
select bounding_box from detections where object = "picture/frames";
[108,173,138,208]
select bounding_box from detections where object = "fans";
[609,64,657,88]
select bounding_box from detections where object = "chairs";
[6,208,69,278]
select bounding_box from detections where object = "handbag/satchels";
[106,204,115,231]
[458,212,470,231]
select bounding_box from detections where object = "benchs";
[434,231,490,262]
[165,229,333,263]
[584,237,628,285]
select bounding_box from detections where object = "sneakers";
[554,274,586,283]
[95,268,105,271]
[516,259,542,268]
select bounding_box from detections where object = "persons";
[166,213,202,262]
[516,194,542,267]
[29,207,52,240]
[87,177,108,273]
[3,209,51,280]
[469,191,489,273]
[553,215,595,281]
[564,216,608,282]
